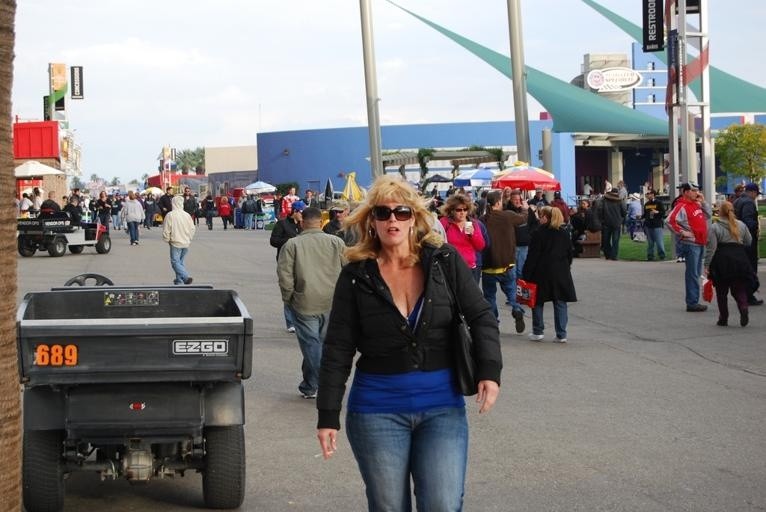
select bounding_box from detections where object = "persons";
[317,172,503,510]
[570,182,667,261]
[418,185,578,344]
[183,187,265,230]
[163,196,196,284]
[19,187,174,246]
[271,184,360,400]
[665,182,764,327]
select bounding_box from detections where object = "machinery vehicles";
[19,211,111,256]
[15,274,253,512]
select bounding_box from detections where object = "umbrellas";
[12,160,66,192]
[492,165,562,201]
[453,168,498,187]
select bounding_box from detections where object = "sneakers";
[289,327,296,332]
[301,390,316,398]
[551,335,569,342]
[740,308,749,329]
[717,319,729,327]
[528,331,545,340]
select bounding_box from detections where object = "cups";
[464,221,473,235]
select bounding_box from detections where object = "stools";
[575,239,601,258]
[628,218,645,240]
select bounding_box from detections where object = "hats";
[629,192,641,201]
[682,181,701,191]
[745,183,764,195]
[535,187,543,192]
[292,202,309,212]
[331,200,350,212]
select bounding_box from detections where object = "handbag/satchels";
[516,277,538,310]
[455,317,481,396]
[123,219,128,230]
[700,274,712,303]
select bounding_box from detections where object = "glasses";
[452,209,470,213]
[372,204,413,222]
[335,210,345,214]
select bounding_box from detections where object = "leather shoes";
[748,297,763,305]
[516,312,525,332]
[688,304,708,313]
